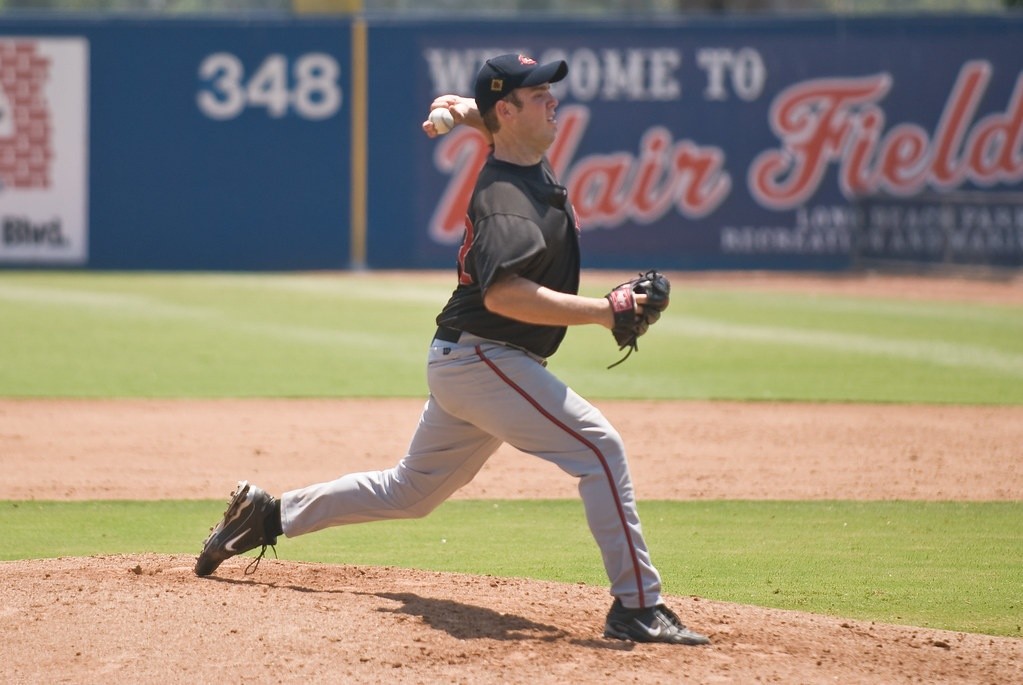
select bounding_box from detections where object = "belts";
[434,324,462,343]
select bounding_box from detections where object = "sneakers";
[194,481,278,576]
[603,598,711,646]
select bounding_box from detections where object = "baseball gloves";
[605,268,670,371]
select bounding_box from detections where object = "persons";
[192,54,712,645]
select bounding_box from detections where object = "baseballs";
[428,107,454,135]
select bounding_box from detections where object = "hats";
[474,52,570,116]
